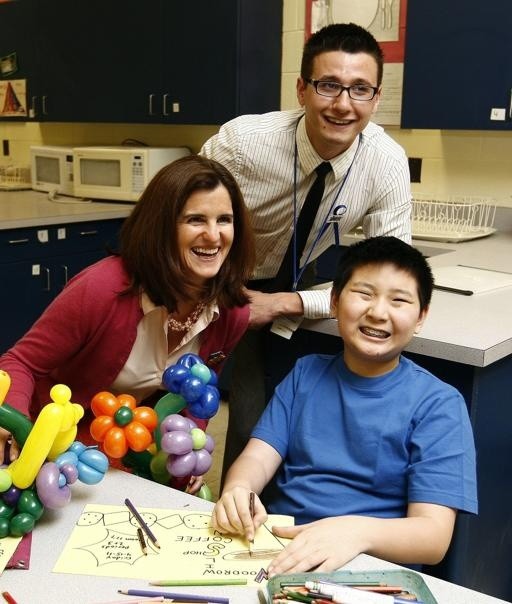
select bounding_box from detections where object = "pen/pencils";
[117,590,230,604]
[250,493,255,557]
[149,580,247,586]
[124,498,160,549]
[137,528,148,557]
[273,582,425,604]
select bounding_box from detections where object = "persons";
[210,237,478,580]
[0,156,257,494]
[197,25,412,502]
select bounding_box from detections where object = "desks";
[0,466,511,604]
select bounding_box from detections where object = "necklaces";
[168,290,209,332]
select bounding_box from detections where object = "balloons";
[0,370,109,539]
[90,352,220,484]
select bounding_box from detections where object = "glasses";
[306,79,379,101]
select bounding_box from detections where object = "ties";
[269,162,334,293]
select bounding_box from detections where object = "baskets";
[411,198,497,234]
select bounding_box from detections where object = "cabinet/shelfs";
[105,1,286,126]
[1,190,138,355]
[401,0,512,132]
[0,0,107,123]
